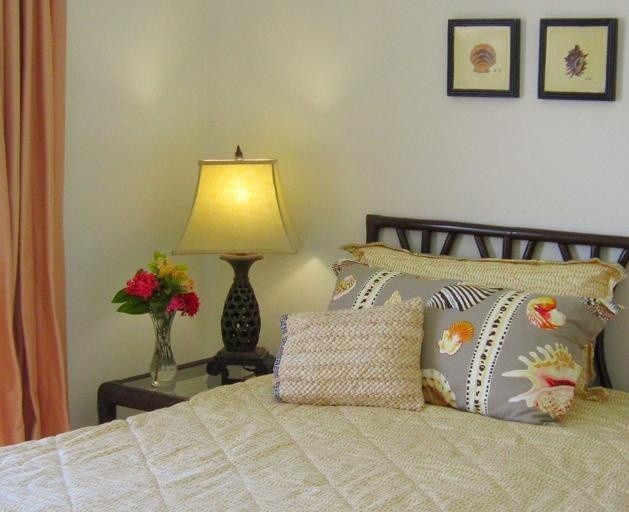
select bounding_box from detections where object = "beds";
[1,214,629,511]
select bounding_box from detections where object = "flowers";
[110,251,200,373]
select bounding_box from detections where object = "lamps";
[171,158,302,373]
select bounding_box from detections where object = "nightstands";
[95,355,274,421]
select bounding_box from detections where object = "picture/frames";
[446,17,521,98]
[536,17,619,102]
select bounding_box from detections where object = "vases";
[148,309,178,384]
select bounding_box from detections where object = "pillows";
[328,260,625,423]
[273,298,424,412]
[339,242,629,295]
